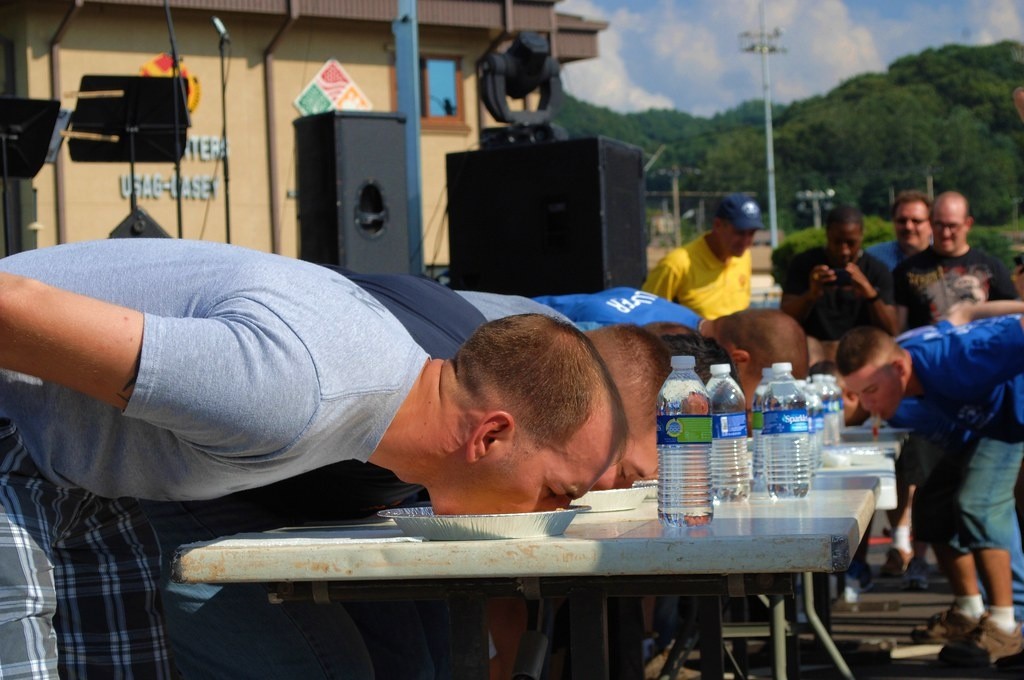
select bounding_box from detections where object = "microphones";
[211,15,231,43]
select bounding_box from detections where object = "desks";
[171,428,911,680]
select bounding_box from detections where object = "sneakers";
[937,611,1023,668]
[910,605,979,645]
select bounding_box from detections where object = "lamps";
[475,29,569,147]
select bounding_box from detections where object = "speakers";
[446,135,649,300]
[293,110,411,278]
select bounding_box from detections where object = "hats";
[720,195,765,231]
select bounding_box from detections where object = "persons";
[781,205,907,343]
[643,195,765,320]
[0,239,1024,680]
[862,190,1024,331]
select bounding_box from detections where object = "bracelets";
[866,288,883,300]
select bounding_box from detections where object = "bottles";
[814,374,846,448]
[655,356,714,529]
[751,368,773,493]
[705,363,751,502]
[798,380,823,475]
[762,362,811,500]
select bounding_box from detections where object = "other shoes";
[881,548,915,575]
[901,557,930,590]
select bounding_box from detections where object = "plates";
[377,505,592,542]
[841,426,912,442]
[822,446,884,465]
[633,480,659,499]
[570,485,653,512]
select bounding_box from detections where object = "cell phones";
[822,269,854,286]
